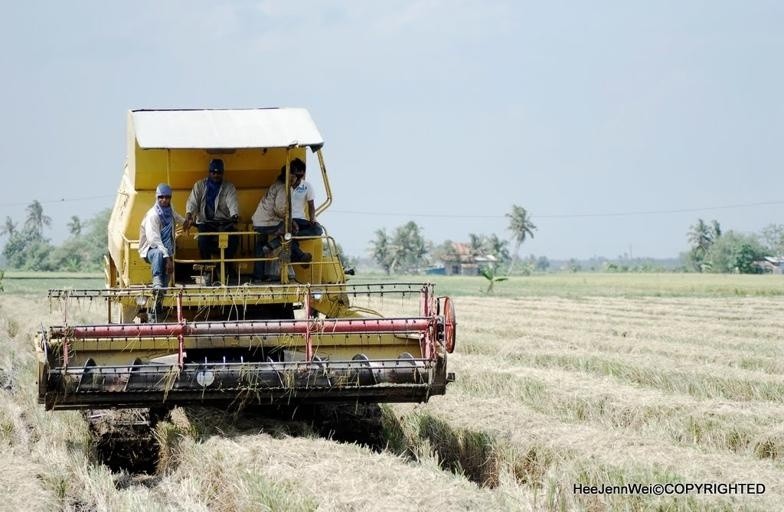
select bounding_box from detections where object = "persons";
[261,158,323,271]
[183,156,243,285]
[250,165,299,283]
[138,182,187,323]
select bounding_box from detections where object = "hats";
[155,183,172,197]
[207,158,225,174]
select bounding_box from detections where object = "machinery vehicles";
[32,104,457,478]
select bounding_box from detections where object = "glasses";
[209,172,221,175]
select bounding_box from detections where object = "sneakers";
[262,244,273,265]
[253,275,272,283]
[299,250,312,269]
